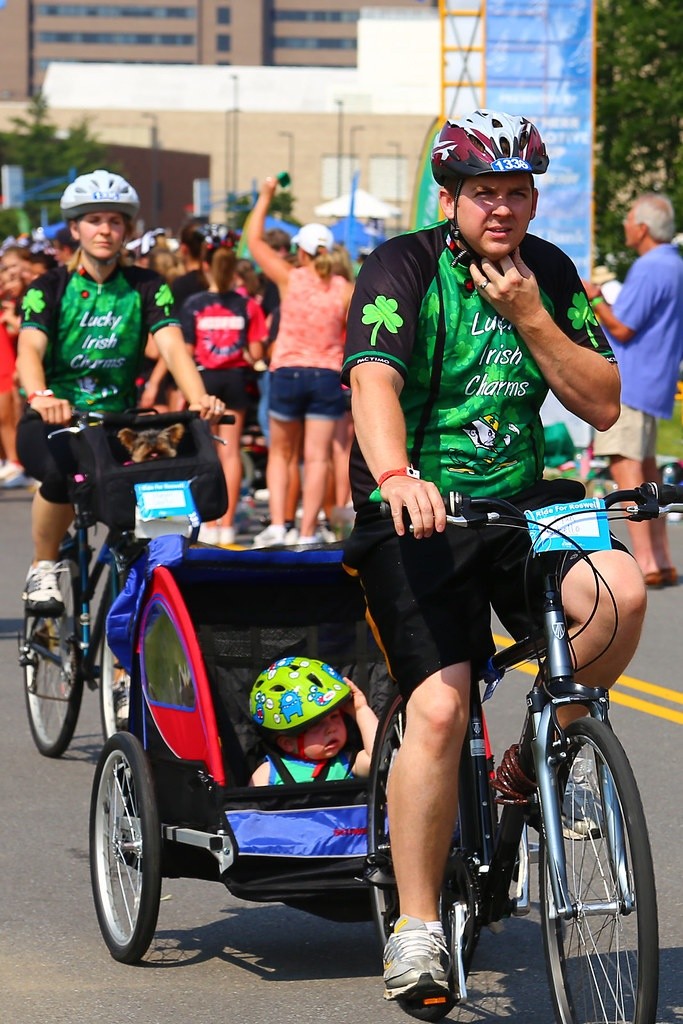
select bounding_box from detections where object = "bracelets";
[27,389,53,404]
[590,297,605,308]
[370,467,419,502]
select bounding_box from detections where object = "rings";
[480,279,489,290]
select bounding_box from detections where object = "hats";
[290,223,334,256]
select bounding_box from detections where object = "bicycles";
[353,463,683,1024]
[14,409,237,758]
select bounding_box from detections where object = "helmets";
[60,170,139,219]
[249,655,355,734]
[431,108,549,186]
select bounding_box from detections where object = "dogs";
[116,423,184,462]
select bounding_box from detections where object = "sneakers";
[21,559,67,615]
[381,923,454,1002]
[552,769,606,843]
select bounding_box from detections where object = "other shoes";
[254,523,293,546]
[286,529,319,552]
[643,565,679,588]
[199,523,238,547]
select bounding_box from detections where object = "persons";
[249,658,383,791]
[341,107,648,997]
[1,169,683,723]
